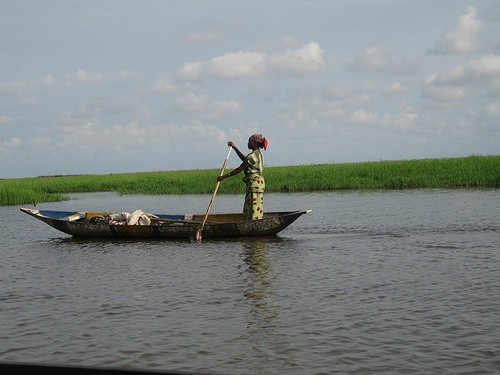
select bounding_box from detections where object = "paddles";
[190,145,233,244]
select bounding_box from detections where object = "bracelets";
[228,173,231,177]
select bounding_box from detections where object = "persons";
[217,134,269,220]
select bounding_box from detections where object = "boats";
[20,208,311,238]
